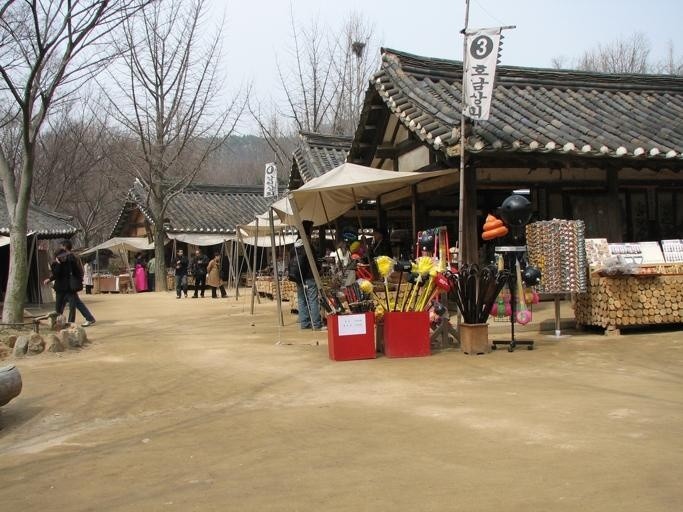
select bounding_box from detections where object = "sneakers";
[82,318,96,327]
[301,324,328,333]
[174,292,229,300]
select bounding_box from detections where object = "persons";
[170,248,189,299]
[133,251,149,292]
[50,248,68,316]
[188,251,195,275]
[188,249,209,298]
[43,238,97,327]
[289,220,327,331]
[83,258,94,295]
[145,253,156,292]
[371,227,394,259]
[315,227,335,257]
[206,251,229,298]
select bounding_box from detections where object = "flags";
[460,27,504,123]
[262,162,278,198]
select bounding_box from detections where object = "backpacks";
[287,255,307,283]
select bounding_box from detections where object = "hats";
[349,241,362,253]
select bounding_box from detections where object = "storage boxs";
[324,311,432,361]
